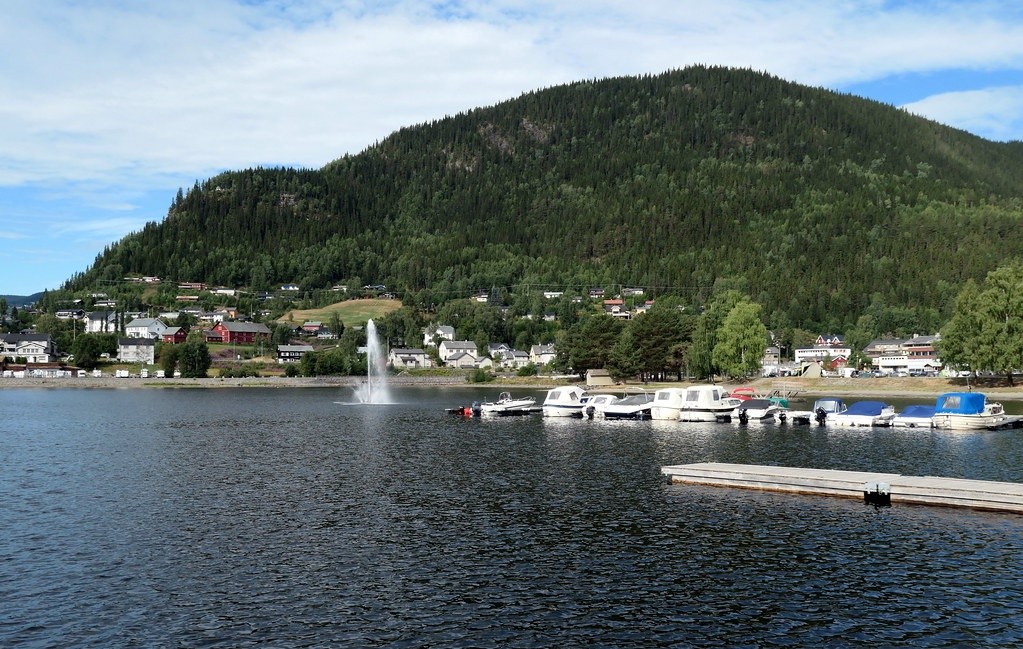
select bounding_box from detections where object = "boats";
[580,394,622,417]
[478,392,536,414]
[603,385,655,421]
[680,384,741,423]
[930,389,1023,428]
[808,395,848,426]
[650,386,688,419]
[893,405,937,428]
[543,386,591,417]
[773,410,812,427]
[833,399,895,428]
[729,395,783,421]
